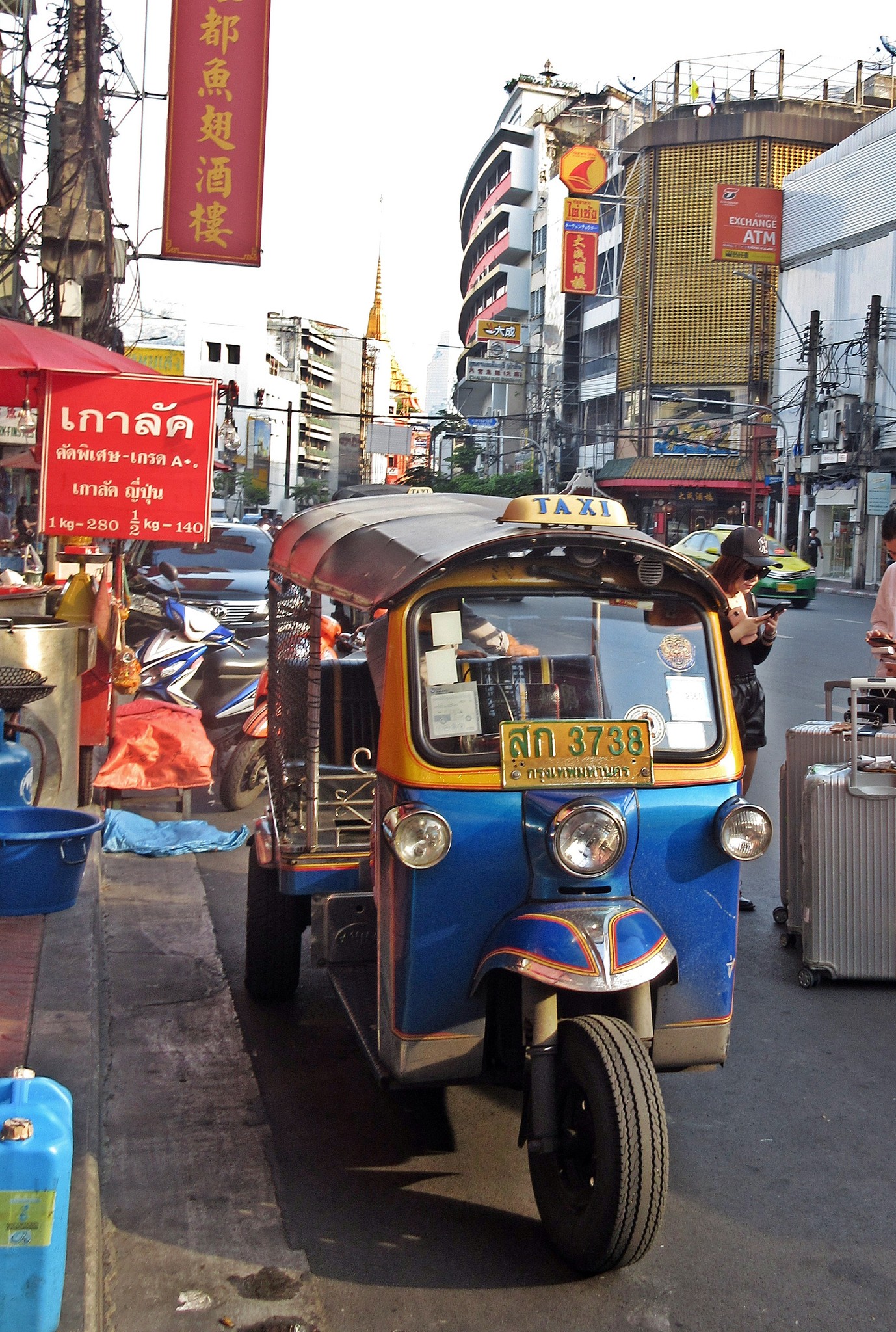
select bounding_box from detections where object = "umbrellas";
[0,319,160,408]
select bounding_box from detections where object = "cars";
[241,513,263,525]
[113,522,281,723]
[669,524,819,609]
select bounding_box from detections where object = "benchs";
[283,654,599,773]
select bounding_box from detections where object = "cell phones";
[869,637,895,644]
[728,607,758,645]
[762,602,789,618]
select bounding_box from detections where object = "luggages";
[799,677,896,989]
[773,680,896,947]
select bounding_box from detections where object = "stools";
[101,718,198,822]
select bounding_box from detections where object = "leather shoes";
[738,894,756,909]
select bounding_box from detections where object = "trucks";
[209,498,229,522]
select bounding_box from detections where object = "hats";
[721,526,783,569]
[809,527,818,534]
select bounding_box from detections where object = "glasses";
[742,562,771,582]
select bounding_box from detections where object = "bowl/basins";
[0,805,106,916]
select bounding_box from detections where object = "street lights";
[733,268,821,558]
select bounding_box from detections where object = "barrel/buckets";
[0,1065,73,1331]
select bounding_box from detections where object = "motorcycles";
[128,558,283,812]
[245,492,776,1271]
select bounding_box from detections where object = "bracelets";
[762,630,777,642]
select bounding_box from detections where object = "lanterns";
[661,502,677,515]
[727,506,741,517]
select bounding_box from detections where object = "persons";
[705,526,786,797]
[808,527,823,567]
[864,508,896,723]
[365,597,539,753]
[0,494,40,539]
[792,536,798,552]
